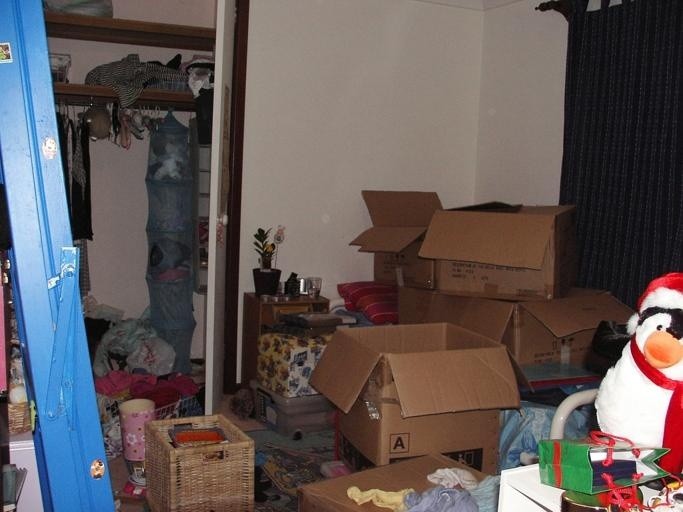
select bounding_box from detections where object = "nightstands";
[241,293,329,386]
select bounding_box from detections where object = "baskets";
[8,403,31,434]
[96,393,200,425]
[143,414,256,512]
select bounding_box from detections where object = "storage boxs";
[395,287,635,367]
[298,452,501,512]
[347,190,577,303]
[310,323,520,475]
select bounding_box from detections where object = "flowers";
[253,228,275,271]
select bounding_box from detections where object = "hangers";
[57,98,163,139]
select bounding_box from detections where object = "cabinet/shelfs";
[44,11,215,104]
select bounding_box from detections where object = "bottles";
[298,278,306,292]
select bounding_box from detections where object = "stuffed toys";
[596,273,683,477]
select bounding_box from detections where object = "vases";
[253,269,281,297]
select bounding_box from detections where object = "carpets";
[126,428,341,512]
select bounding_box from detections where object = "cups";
[117,399,156,487]
[307,276,322,298]
[49,51,72,86]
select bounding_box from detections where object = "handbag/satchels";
[538,431,671,496]
